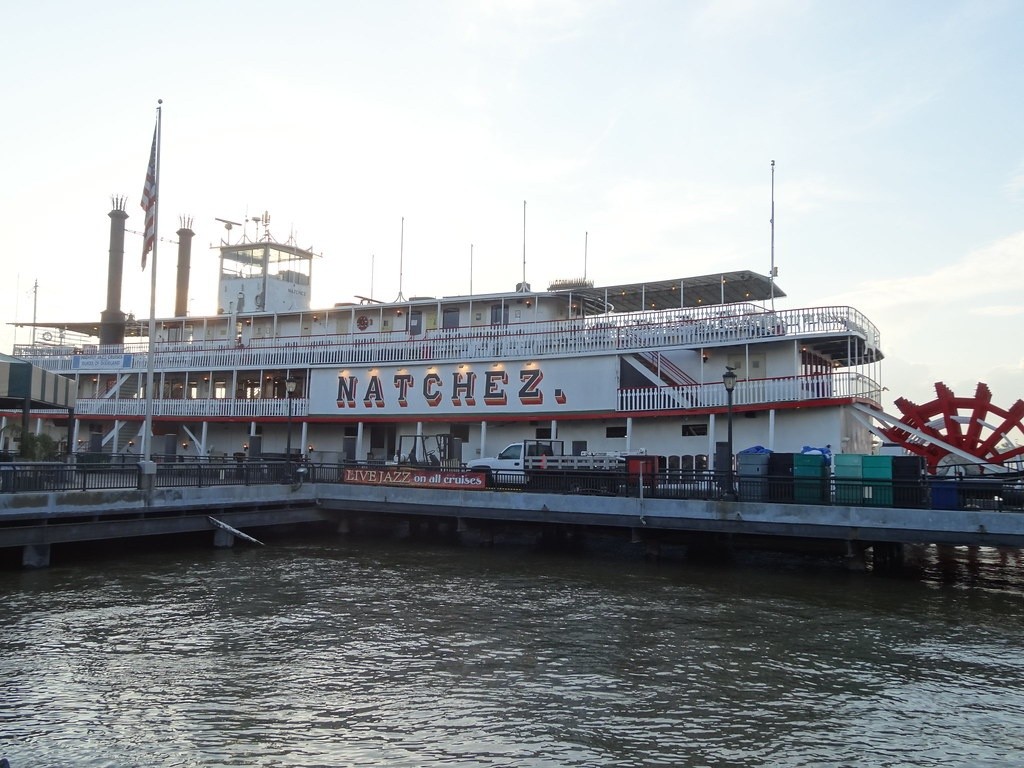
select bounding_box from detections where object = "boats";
[1,98,1024,491]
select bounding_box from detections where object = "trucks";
[467,439,626,494]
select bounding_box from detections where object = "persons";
[140,115,157,272]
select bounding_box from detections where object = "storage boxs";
[931,481,959,510]
[735,453,924,508]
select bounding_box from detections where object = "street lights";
[723,365,740,500]
[281,375,296,484]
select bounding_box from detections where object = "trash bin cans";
[768,452,793,503]
[891,456,928,505]
[834,453,862,503]
[793,454,831,504]
[861,455,892,505]
[928,482,964,510]
[737,453,768,502]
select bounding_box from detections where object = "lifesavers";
[42,332,53,341]
